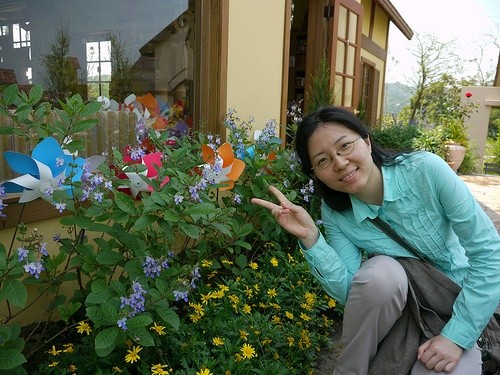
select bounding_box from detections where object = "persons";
[250,106,500,374]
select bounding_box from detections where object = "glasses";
[310,134,361,170]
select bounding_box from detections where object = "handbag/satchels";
[478,313,500,375]
